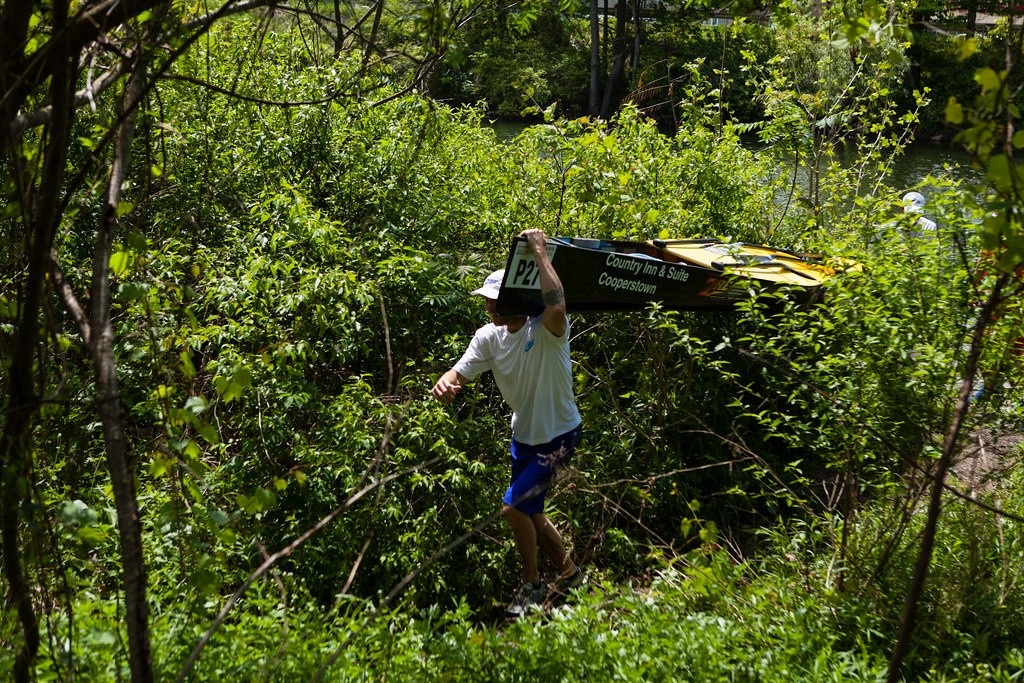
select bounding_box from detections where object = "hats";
[470,269,506,300]
[903,192,924,215]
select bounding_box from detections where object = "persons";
[431,228,583,624]
[868,192,937,254]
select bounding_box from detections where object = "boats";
[895,340,987,413]
[968,247,1024,355]
[494,228,867,318]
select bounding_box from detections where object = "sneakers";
[504,581,548,622]
[549,567,584,602]
